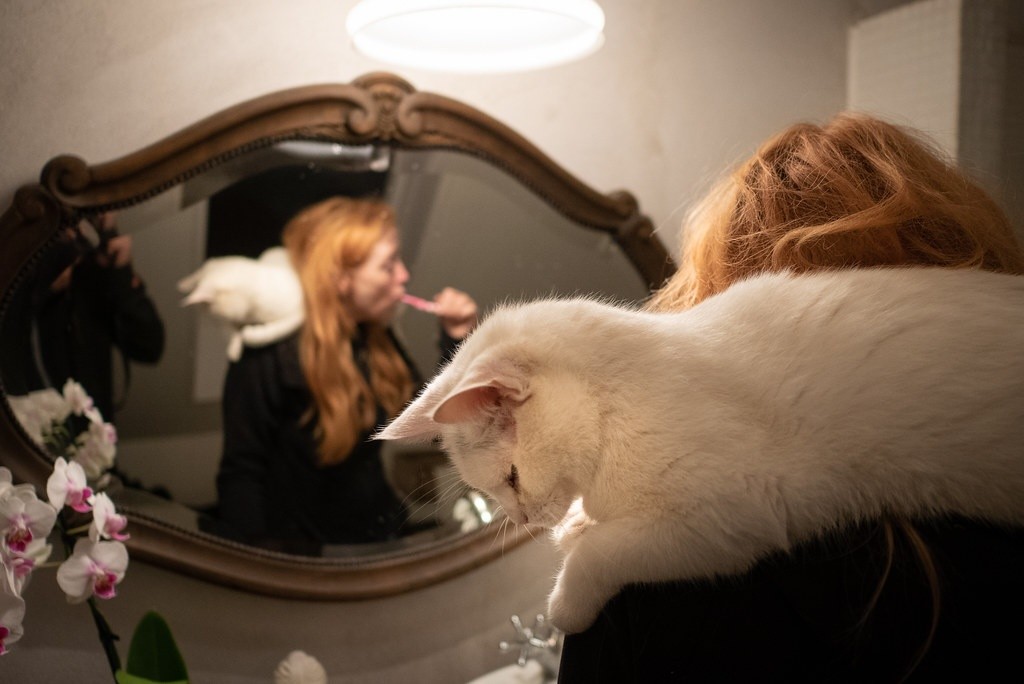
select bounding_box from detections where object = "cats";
[177,248,308,362]
[374,256,1023,632]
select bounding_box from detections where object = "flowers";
[6,377,117,488]
[0,457,326,684]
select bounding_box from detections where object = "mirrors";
[0,70,679,605]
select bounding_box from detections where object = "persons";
[36,211,175,504]
[215,195,476,556]
[557,118,1024,684]
